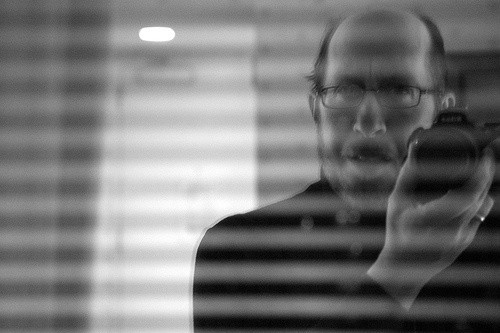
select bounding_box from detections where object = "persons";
[192,10,500,333]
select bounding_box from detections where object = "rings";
[475,214,485,222]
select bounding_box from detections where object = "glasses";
[317,85,442,109]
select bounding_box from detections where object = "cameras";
[408,110,500,193]
